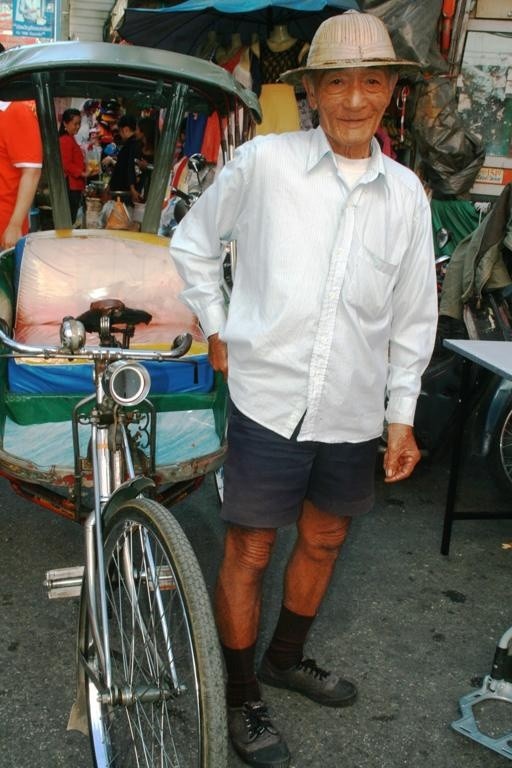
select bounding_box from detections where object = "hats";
[279,6,422,89]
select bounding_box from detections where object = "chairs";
[7,229,214,395]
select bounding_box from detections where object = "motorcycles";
[400,181,512,494]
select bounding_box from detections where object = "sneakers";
[257,642,359,708]
[225,689,292,767]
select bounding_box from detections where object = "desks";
[441,338,512,556]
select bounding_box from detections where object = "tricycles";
[0,38,262,767]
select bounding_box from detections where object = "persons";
[184,22,311,168]
[2,41,42,255]
[19,0,41,24]
[58,91,162,230]
[167,7,437,767]
